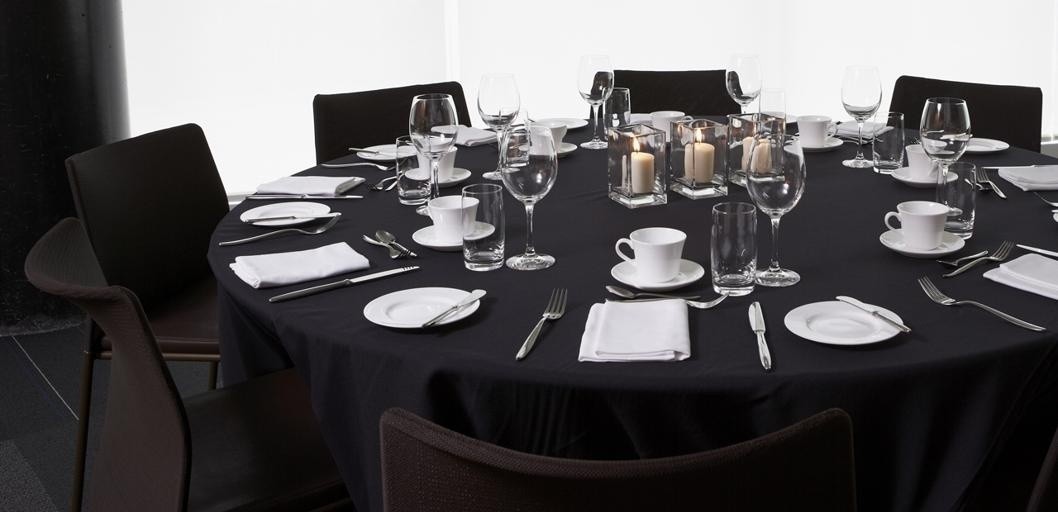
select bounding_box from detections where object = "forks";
[916,273,1047,336]
[361,235,412,260]
[219,213,342,252]
[935,251,988,267]
[620,292,730,309]
[943,241,1015,278]
[511,283,571,358]
[974,167,1007,200]
[320,160,405,172]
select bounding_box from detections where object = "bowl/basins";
[616,228,688,282]
[796,115,838,145]
[527,119,569,151]
[903,144,931,177]
[427,195,478,236]
[884,201,949,249]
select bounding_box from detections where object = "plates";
[413,222,495,250]
[788,136,845,151]
[608,259,707,292]
[878,228,965,257]
[890,167,960,189]
[967,136,1009,153]
[782,303,905,344]
[536,116,590,132]
[518,141,576,157]
[365,288,480,328]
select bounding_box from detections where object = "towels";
[828,120,894,139]
[256,175,366,197]
[982,253,1058,301]
[577,298,692,362]
[998,164,1058,192]
[612,113,653,128]
[228,240,371,290]
[430,124,498,148]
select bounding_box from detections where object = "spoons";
[377,230,420,260]
[604,284,704,301]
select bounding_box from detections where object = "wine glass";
[842,66,883,170]
[477,74,523,174]
[577,57,614,150]
[746,133,808,288]
[725,62,763,116]
[921,95,971,217]
[500,126,559,271]
[408,92,460,216]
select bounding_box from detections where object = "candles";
[684,141,715,184]
[621,150,656,194]
[741,136,772,174]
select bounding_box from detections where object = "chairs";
[65,123,230,512]
[313,82,472,166]
[886,75,1042,153]
[590,69,742,119]
[23,219,352,511]
[379,407,857,512]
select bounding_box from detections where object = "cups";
[670,118,730,202]
[395,136,433,206]
[764,88,786,136]
[605,86,631,132]
[727,112,788,188]
[461,183,507,271]
[872,111,905,175]
[650,111,693,142]
[709,202,759,298]
[605,123,670,210]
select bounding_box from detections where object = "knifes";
[267,266,420,301]
[243,193,366,201]
[747,299,773,371]
[833,293,910,334]
[418,287,490,326]
